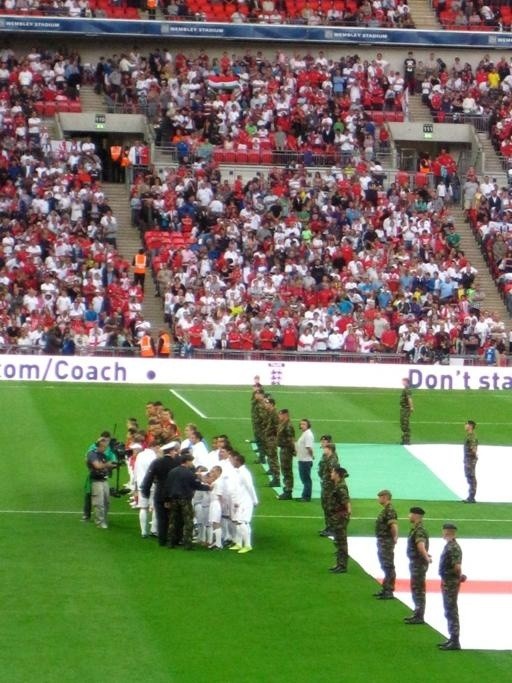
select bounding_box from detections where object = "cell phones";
[109,438,133,461]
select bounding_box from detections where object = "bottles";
[263,398,275,405]
[441,523,457,532]
[279,408,289,414]
[376,489,391,497]
[160,440,181,454]
[253,374,260,379]
[408,507,426,516]
[465,419,475,426]
[252,389,265,395]
[401,377,409,383]
[250,383,261,389]
[322,443,336,450]
[95,437,109,444]
[321,433,332,441]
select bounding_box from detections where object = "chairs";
[79,515,89,522]
[140,533,146,539]
[237,545,253,554]
[461,498,476,504]
[97,523,108,529]
[130,505,140,509]
[123,484,128,486]
[149,531,158,538]
[128,496,135,500]
[199,541,209,547]
[228,544,241,551]
[125,486,131,490]
[400,441,409,445]
[207,544,220,550]
[128,501,136,506]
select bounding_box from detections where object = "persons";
[2,138,113,253]
[131,442,161,540]
[263,400,279,488]
[251,390,268,465]
[163,424,183,453]
[250,377,262,399]
[402,51,416,93]
[220,454,247,543]
[326,468,350,572]
[212,435,229,506]
[229,456,260,552]
[141,441,179,545]
[153,400,165,420]
[87,438,116,528]
[131,250,147,290]
[110,141,122,180]
[157,330,171,358]
[179,424,205,459]
[146,416,161,443]
[203,466,224,552]
[321,444,340,538]
[150,140,511,364]
[316,437,335,477]
[400,379,414,444]
[153,421,167,446]
[125,429,146,491]
[191,465,214,546]
[2,0,511,34]
[124,140,156,243]
[141,332,154,358]
[438,522,467,649]
[2,43,83,138]
[160,409,175,427]
[161,455,210,548]
[464,420,478,505]
[175,320,511,367]
[81,431,116,523]
[122,419,137,447]
[372,490,399,599]
[295,418,314,502]
[212,446,236,533]
[187,433,209,470]
[404,507,431,625]
[276,409,296,500]
[144,402,157,418]
[3,245,129,355]
[93,46,406,135]
[414,52,511,155]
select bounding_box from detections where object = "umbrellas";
[184,546,196,552]
[253,448,259,453]
[440,642,461,652]
[227,542,235,547]
[276,494,284,497]
[403,615,414,620]
[330,566,348,574]
[262,481,281,488]
[376,595,394,600]
[405,618,425,626]
[223,539,231,546]
[248,439,257,444]
[157,540,168,547]
[320,532,333,538]
[264,469,272,476]
[318,529,326,533]
[253,458,268,464]
[328,565,337,570]
[437,640,448,647]
[278,496,292,501]
[296,498,309,503]
[372,591,383,596]
[167,543,175,550]
